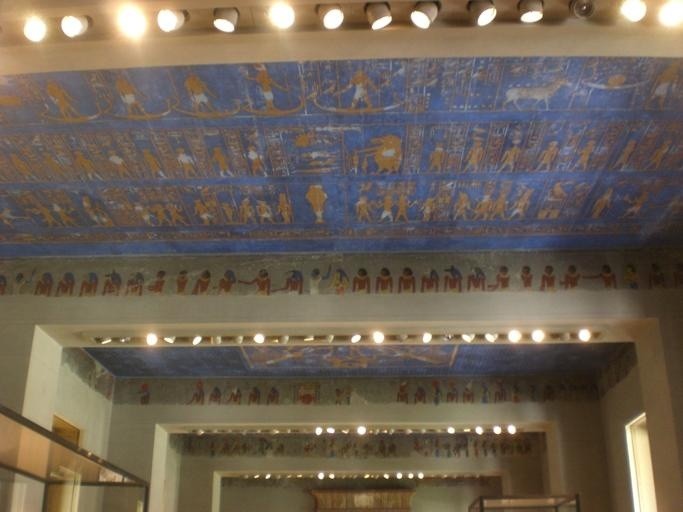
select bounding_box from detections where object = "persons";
[136,383,150,404]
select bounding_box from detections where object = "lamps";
[23,0,683,46]
